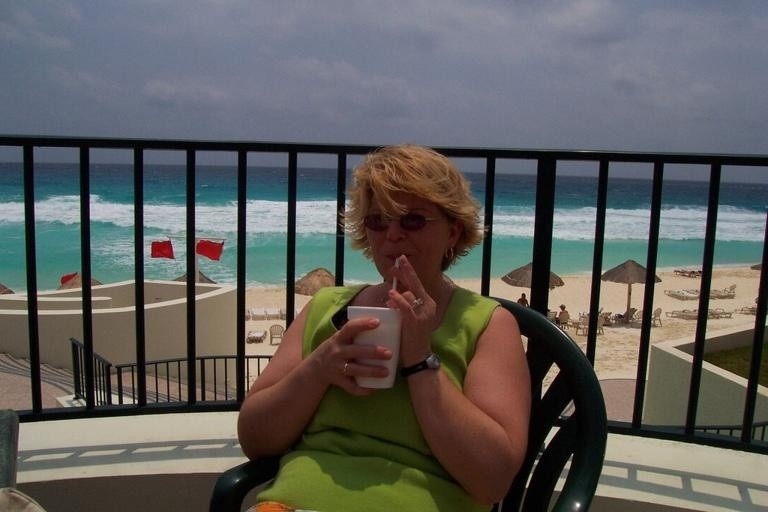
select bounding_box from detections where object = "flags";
[61,272,77,284]
[196,239,224,261]
[151,240,174,259]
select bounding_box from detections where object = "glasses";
[363,215,444,232]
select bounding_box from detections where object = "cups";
[348,306,403,390]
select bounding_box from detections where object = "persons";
[555,304,569,325]
[517,293,529,308]
[235,140,534,511]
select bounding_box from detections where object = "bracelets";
[399,350,444,381]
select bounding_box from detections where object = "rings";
[409,297,424,308]
[342,362,352,376]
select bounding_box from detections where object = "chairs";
[676,270,703,277]
[246,306,306,345]
[665,287,736,300]
[549,305,758,339]
[211,298,608,511]
